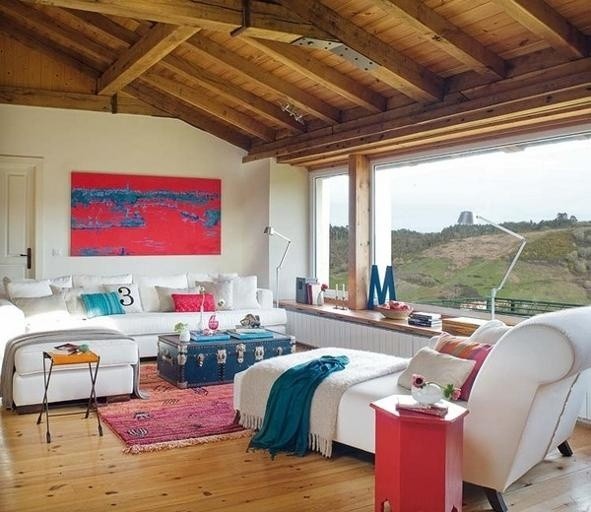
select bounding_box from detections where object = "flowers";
[410,372,462,402]
[172,321,189,331]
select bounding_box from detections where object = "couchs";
[233,306,591,512]
[0,271,287,414]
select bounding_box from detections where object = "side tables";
[370,394,471,510]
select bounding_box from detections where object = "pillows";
[11,292,71,327]
[104,284,143,313]
[433,331,494,401]
[3,278,52,299]
[196,281,233,311]
[155,284,201,312]
[219,276,261,309]
[397,346,477,399]
[81,292,126,319]
[466,319,513,345]
[170,293,215,312]
[49,284,106,314]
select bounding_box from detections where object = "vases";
[180,331,190,342]
[411,385,442,408]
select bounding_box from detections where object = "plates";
[379,307,413,320]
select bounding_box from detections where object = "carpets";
[88,362,259,457]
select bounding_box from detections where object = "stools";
[37,347,104,444]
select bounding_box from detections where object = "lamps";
[455,211,527,321]
[264,227,292,308]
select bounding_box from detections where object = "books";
[226,329,274,340]
[311,284,322,305]
[234,325,265,334]
[190,330,230,341]
[408,312,441,329]
[307,282,320,305]
[397,397,448,418]
